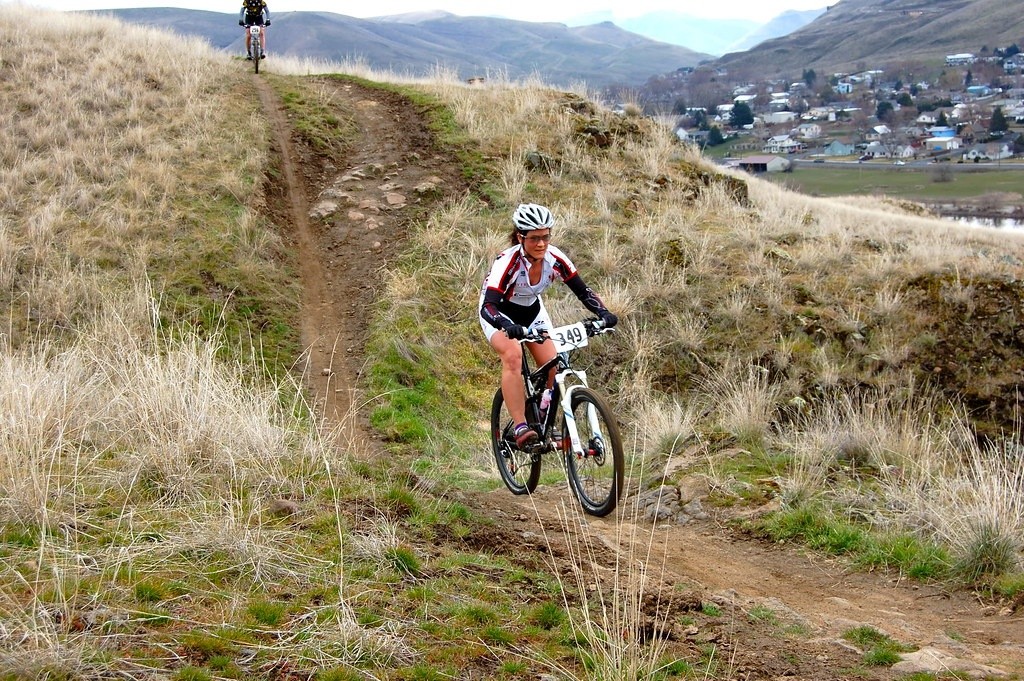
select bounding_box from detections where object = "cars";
[894,160,906,166]
[858,155,873,161]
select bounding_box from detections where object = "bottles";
[539,389,552,418]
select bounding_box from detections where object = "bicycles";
[239,20,271,74]
[489,317,626,516]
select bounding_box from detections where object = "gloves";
[265,19,271,27]
[239,20,244,26]
[502,322,524,342]
[600,311,617,328]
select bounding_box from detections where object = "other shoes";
[260,52,265,59]
[247,53,251,60]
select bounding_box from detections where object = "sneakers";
[513,422,537,448]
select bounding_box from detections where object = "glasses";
[517,230,553,243]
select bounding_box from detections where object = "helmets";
[513,203,554,231]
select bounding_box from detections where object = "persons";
[478,203,618,448]
[239,0,271,60]
